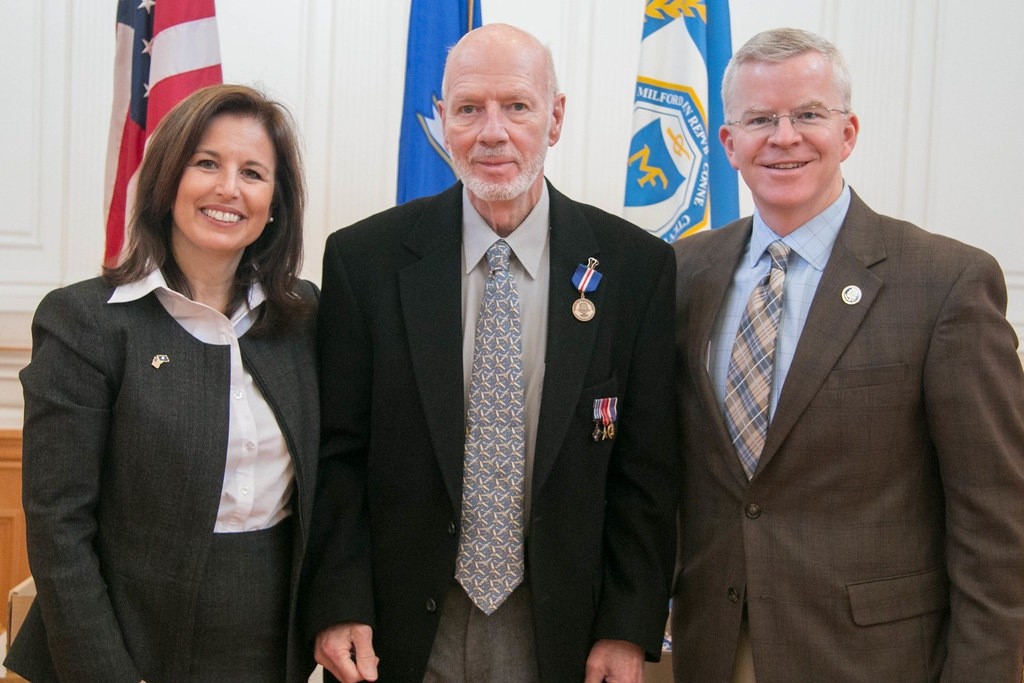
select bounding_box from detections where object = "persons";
[669,25,1023,683]
[2,83,322,683]
[308,22,683,683]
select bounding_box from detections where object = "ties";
[454,239,524,617]
[723,241,794,482]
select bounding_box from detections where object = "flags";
[99,1,223,275]
[617,0,740,248]
[393,1,483,207]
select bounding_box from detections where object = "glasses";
[725,106,848,136]
